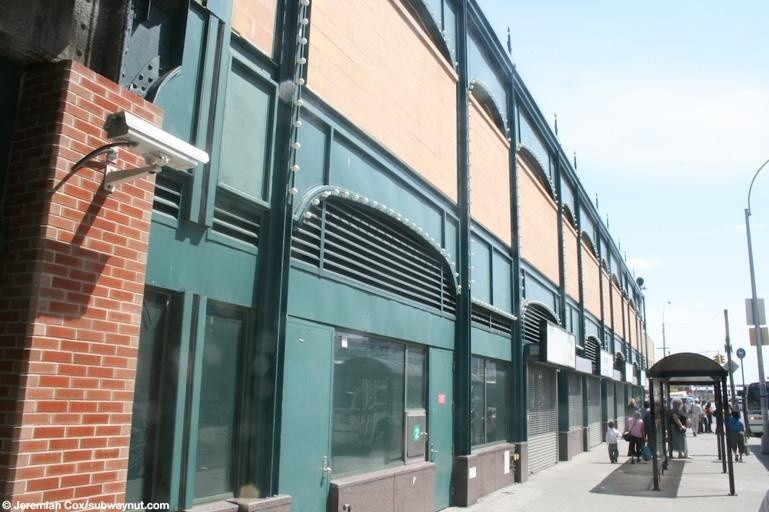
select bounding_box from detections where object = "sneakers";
[735,455,743,462]
[611,458,617,463]
[678,456,689,459]
[631,459,641,464]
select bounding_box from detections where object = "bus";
[746,381,769,437]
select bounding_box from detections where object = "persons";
[605,398,651,465]
[470,403,497,446]
[668,396,750,464]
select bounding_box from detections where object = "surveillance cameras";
[103,110,217,171]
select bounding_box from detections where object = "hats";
[732,411,740,417]
[644,399,650,406]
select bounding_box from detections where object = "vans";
[471,380,497,442]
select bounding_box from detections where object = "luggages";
[641,447,651,461]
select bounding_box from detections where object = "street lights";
[661,299,672,357]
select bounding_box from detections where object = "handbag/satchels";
[623,430,631,441]
[679,414,688,428]
